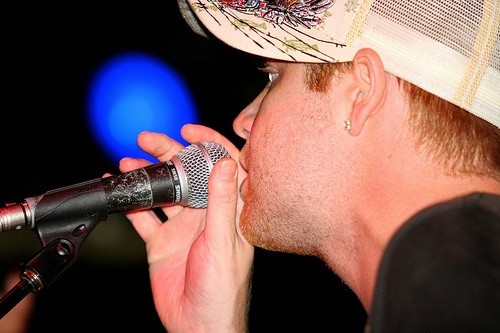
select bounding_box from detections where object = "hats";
[178,0,499,127]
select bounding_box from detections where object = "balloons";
[87,52,197,165]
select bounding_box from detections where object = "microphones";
[0,143,233,233]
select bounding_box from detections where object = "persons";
[103,0,500,333]
